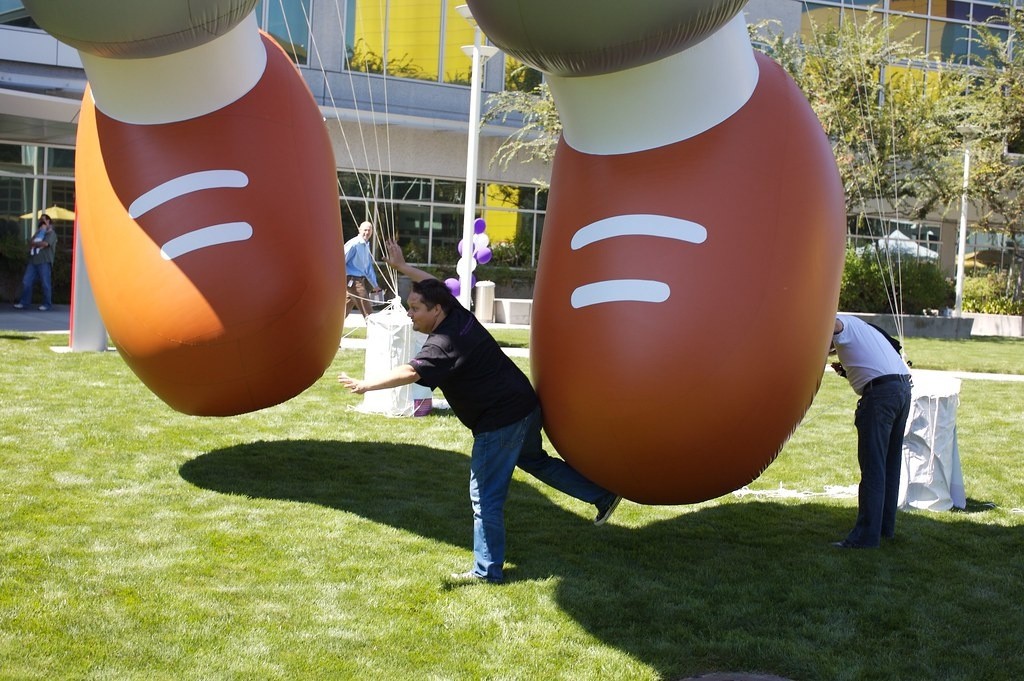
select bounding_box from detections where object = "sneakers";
[595,497,622,525]
[450,572,481,583]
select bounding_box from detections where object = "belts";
[862,374,910,391]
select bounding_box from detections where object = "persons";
[338,220,383,349]
[13,213,59,312]
[829,312,915,550]
[337,237,624,587]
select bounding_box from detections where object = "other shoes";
[38,306,47,310]
[830,541,847,547]
[14,303,23,309]
